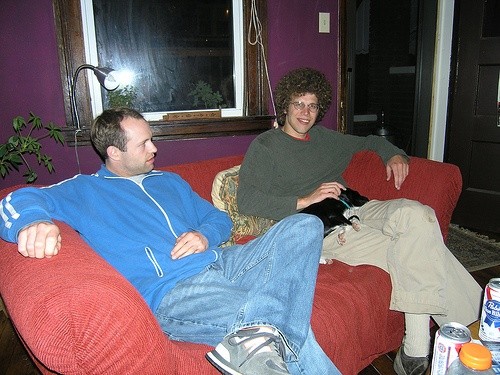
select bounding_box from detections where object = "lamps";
[71,64,122,132]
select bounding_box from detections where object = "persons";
[0,109,343,375]
[237,68,484,375]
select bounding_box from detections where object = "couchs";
[0,149,463,375]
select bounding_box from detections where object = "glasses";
[290,101,322,113]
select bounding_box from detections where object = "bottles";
[378,111,389,142]
[445,343,496,375]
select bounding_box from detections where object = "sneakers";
[205,328,293,375]
[394,343,431,375]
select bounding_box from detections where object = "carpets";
[446,223,500,272]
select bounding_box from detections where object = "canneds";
[430,322,471,375]
[478,278,500,342]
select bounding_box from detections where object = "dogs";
[298,187,370,264]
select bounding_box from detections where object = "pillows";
[211,165,276,237]
